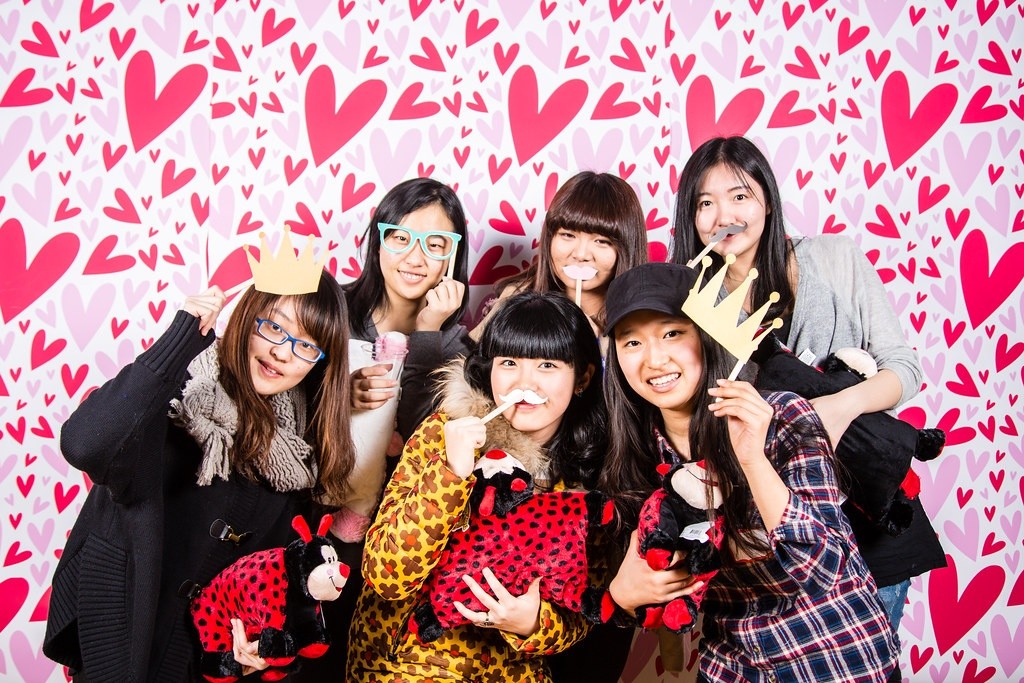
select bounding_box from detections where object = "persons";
[42,269,354,683]
[469,171,647,359]
[330,178,469,567]
[347,293,608,683]
[669,137,923,635]
[603,263,900,683]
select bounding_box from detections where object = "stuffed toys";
[602,458,732,634]
[192,514,350,683]
[406,450,621,648]
[751,327,944,528]
[330,332,408,543]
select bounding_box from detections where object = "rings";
[484,612,494,626]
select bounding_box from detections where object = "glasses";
[377,222,462,279]
[255,318,326,363]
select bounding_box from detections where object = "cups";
[360,343,409,400]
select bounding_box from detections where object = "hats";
[601,262,699,336]
